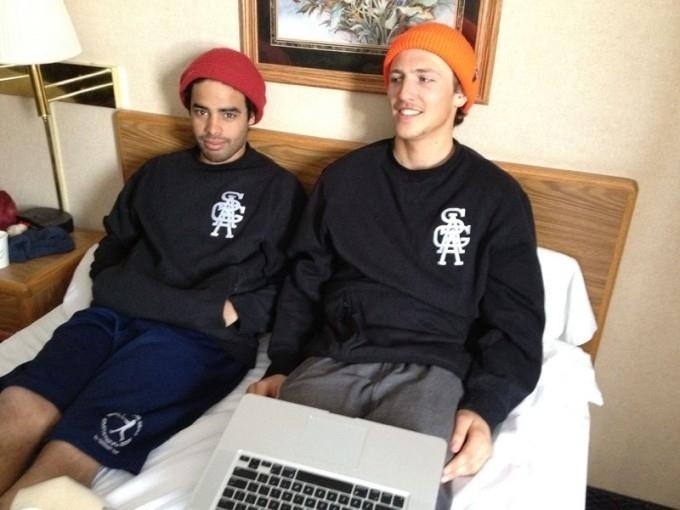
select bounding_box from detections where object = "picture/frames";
[237,0,503,105]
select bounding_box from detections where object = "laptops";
[186,393,447,510]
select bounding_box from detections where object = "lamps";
[0,2,83,233]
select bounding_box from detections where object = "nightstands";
[0,224,108,343]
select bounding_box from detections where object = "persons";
[1,48,309,510]
[247,23,546,510]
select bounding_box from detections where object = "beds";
[0,109,638,509]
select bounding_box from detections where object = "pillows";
[536,247,605,407]
[62,242,99,318]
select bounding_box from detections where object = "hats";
[383,23,477,113]
[179,47,266,125]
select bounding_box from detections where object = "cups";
[0,231,9,269]
